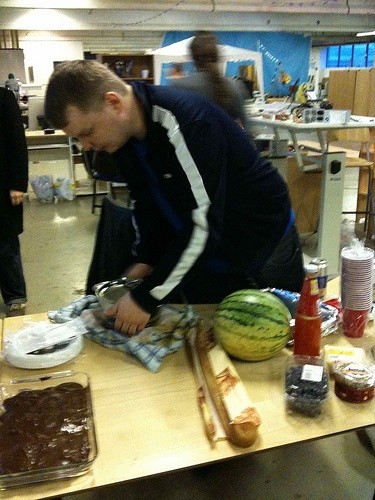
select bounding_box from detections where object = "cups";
[141,69,149,78]
[340,247,374,339]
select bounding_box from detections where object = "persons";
[167,34,254,129]
[0,72,30,315]
[46,60,308,331]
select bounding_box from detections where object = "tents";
[152,37,268,101]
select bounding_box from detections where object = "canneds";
[309,257,328,298]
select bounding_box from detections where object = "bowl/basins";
[284,355,330,420]
[333,360,375,403]
[0,370,98,491]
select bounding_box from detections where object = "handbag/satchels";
[31,174,54,203]
[53,176,75,200]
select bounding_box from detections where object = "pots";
[92,274,166,333]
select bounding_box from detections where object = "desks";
[0,275,374,500]
[246,104,375,276]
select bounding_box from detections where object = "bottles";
[294,263,321,361]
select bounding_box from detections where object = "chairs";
[327,126,375,232]
[84,151,116,214]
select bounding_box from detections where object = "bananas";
[298,83,307,103]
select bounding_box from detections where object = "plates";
[6,323,83,369]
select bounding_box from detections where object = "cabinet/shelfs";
[325,70,375,143]
[24,130,127,197]
[101,54,155,85]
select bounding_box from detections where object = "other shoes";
[5,298,27,317]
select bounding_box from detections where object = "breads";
[197,328,256,448]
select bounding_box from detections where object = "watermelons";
[213,290,291,361]
